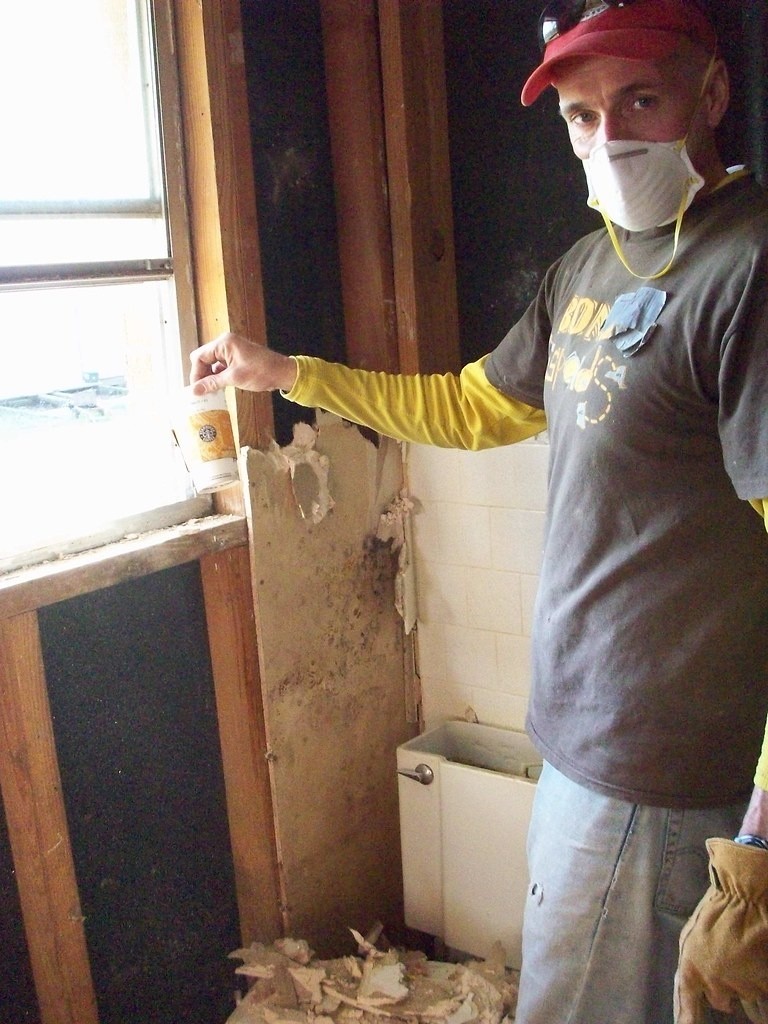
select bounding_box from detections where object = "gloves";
[673,837,768,1024]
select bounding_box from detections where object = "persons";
[189,0,768,1024]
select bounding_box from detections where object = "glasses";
[538,0,636,57]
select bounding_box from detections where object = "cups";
[167,380,242,497]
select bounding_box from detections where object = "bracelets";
[735,834,768,849]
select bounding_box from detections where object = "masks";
[582,35,719,279]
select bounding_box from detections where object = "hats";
[521,0,721,106]
